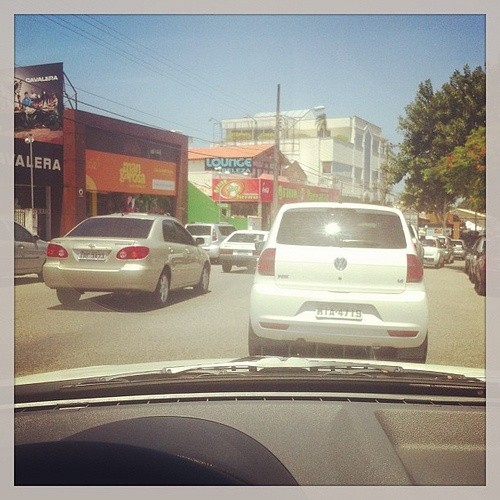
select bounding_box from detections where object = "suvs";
[246,202,431,364]
[184,222,237,259]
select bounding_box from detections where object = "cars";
[219,229,270,274]
[42,212,211,305]
[14,222,51,282]
[409,223,486,296]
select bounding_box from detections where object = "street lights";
[25,134,35,210]
[215,167,231,222]
[271,105,327,228]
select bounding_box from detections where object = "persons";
[14,91,58,110]
